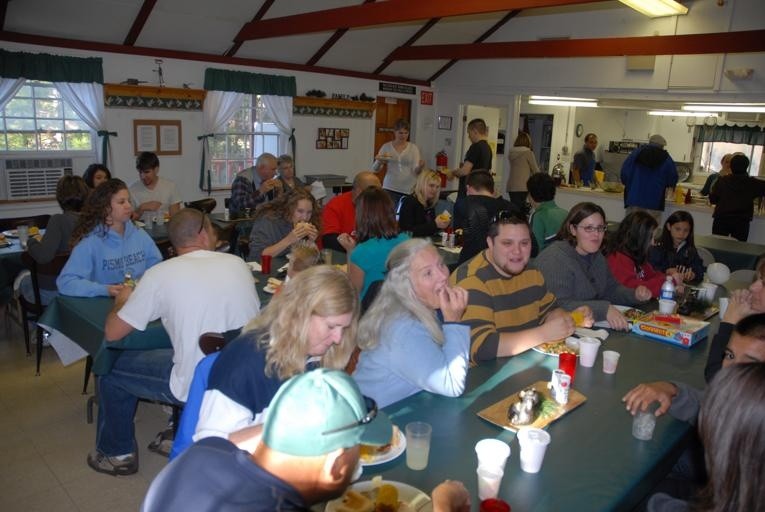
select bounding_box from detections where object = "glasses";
[321,396,378,436]
[186,202,206,234]
[487,208,529,228]
[574,224,608,233]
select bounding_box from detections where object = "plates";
[356,426,406,469]
[261,286,276,294]
[2,229,34,237]
[532,337,581,358]
[610,304,644,322]
[153,216,170,223]
[475,379,587,436]
[323,479,433,512]
[134,221,145,228]
[374,156,392,161]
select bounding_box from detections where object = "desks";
[0,173,765,512]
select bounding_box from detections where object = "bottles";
[441,231,457,248]
[657,276,675,298]
[675,184,692,205]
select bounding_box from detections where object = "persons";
[453,118,493,201]
[506,130,542,217]
[573,134,601,186]
[10,150,763,511]
[372,118,428,208]
[620,133,681,232]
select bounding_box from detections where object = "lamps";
[647,109,722,118]
[527,95,599,108]
[681,102,765,114]
[617,0,689,20]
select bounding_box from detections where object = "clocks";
[576,124,583,137]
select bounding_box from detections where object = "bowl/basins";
[599,181,625,193]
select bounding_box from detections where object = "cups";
[16,225,28,247]
[551,338,620,404]
[260,254,272,275]
[224,207,252,221]
[402,421,434,471]
[320,248,333,266]
[630,400,658,441]
[658,298,676,314]
[142,209,165,230]
[665,187,672,199]
[700,282,730,319]
[474,426,552,512]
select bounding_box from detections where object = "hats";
[260,366,394,458]
[650,134,667,146]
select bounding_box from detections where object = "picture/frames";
[133,120,159,156]
[439,116,452,130]
[316,128,349,149]
[159,120,182,155]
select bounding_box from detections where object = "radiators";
[0,151,97,201]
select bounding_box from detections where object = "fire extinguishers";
[436,150,448,188]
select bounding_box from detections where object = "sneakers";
[86,442,138,475]
[147,430,174,458]
[30,329,50,348]
